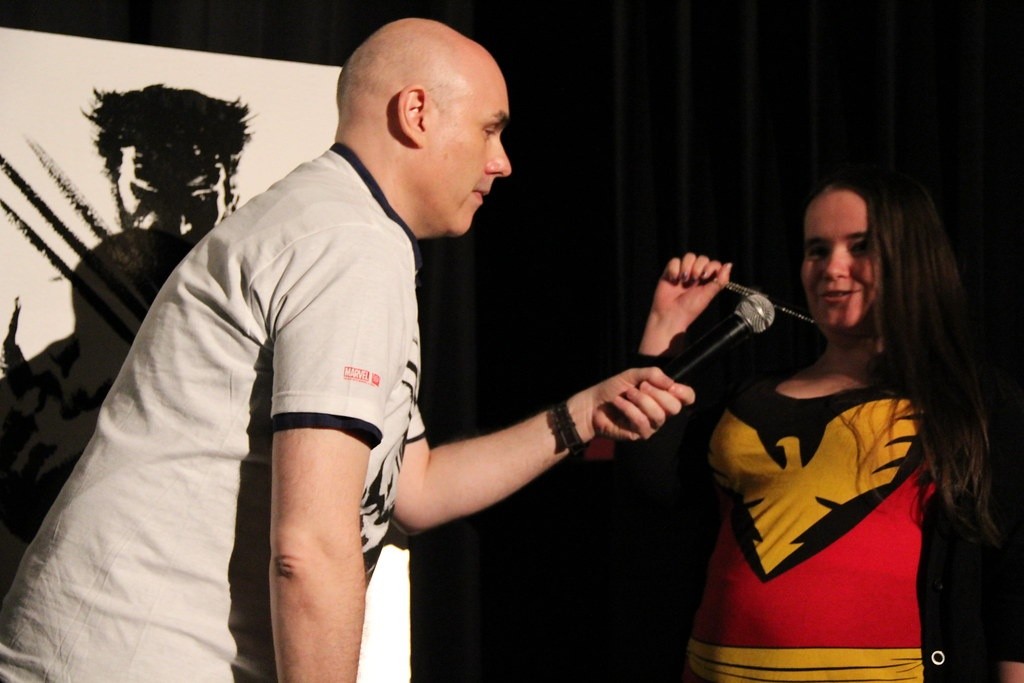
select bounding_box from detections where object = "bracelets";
[550,403,585,455]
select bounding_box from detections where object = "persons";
[619,161,1024,683]
[1,15,697,682]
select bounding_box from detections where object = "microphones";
[635,293,776,390]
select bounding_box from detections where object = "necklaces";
[712,277,814,325]
